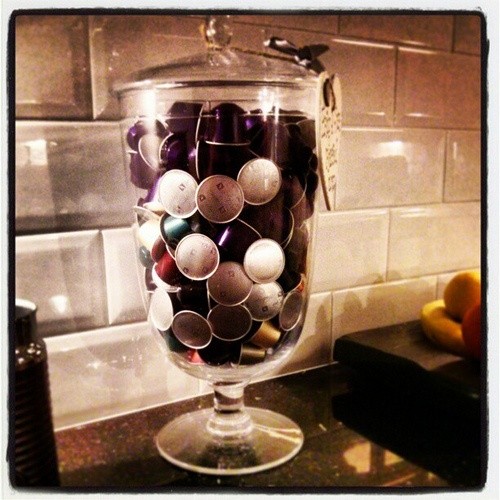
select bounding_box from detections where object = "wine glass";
[113,12,334,476]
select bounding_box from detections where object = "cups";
[14,299,64,484]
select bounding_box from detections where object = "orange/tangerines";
[443,270,481,318]
[461,301,481,358]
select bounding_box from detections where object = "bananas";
[420,299,464,352]
